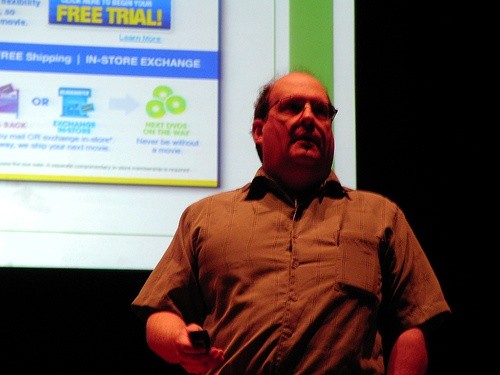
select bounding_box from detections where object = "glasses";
[263,96,338,122]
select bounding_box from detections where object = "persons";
[131,71,452,375]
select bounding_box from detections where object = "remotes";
[190,330,210,353]
